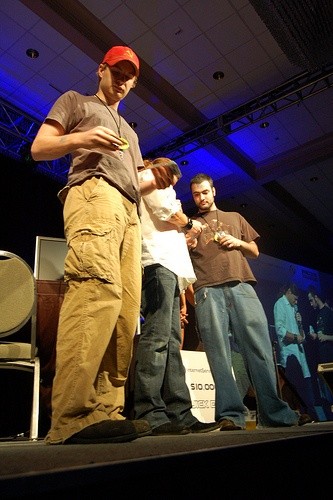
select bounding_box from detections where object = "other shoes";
[221,419,240,430]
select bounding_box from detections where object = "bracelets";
[182,218,193,229]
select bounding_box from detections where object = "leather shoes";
[191,422,219,432]
[65,420,152,442]
[155,423,190,434]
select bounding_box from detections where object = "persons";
[186,174,305,428]
[30,46,178,444]
[122,157,216,434]
[273,284,314,414]
[304,283,333,422]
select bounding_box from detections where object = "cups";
[243,410,256,431]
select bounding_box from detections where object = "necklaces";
[95,93,122,138]
[201,209,219,233]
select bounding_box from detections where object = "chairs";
[0,250,41,440]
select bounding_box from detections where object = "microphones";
[296,311,303,332]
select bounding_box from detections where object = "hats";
[102,46,139,77]
